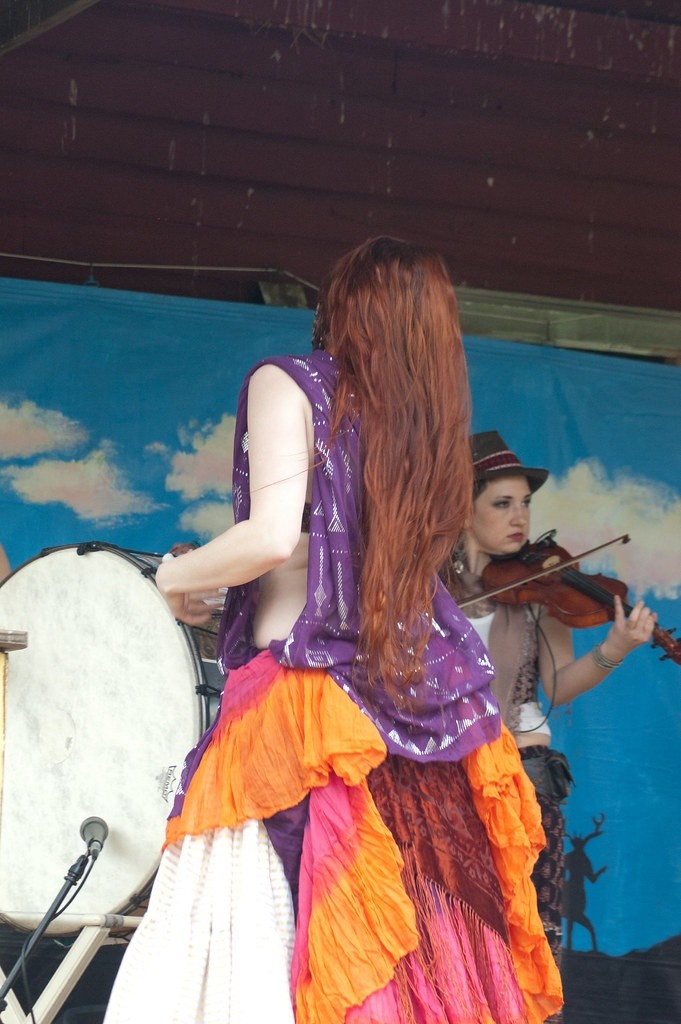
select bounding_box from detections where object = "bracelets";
[591,641,624,670]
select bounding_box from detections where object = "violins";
[482,544,681,665]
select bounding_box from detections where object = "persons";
[438,430,658,1024]
[104,234,564,1024]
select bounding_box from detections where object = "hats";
[468,430,549,493]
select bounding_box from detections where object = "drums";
[0,539,228,938]
